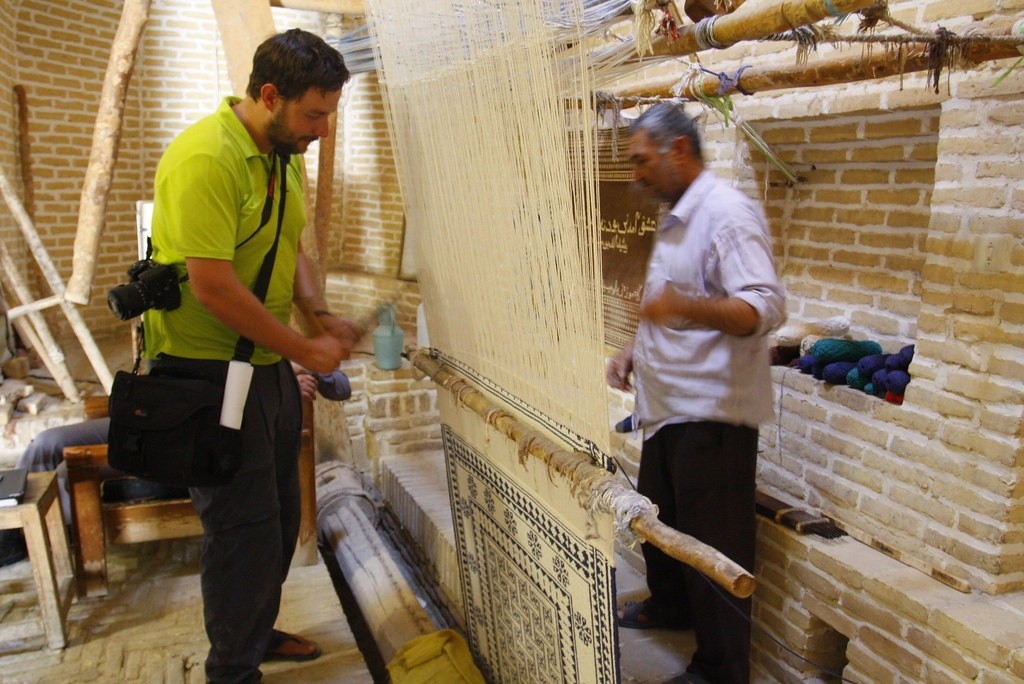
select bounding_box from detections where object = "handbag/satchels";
[107,369,247,489]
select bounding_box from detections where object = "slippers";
[666,673,707,684]
[617,597,689,630]
[263,629,321,662]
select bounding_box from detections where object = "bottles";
[373,304,403,370]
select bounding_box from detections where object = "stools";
[0,470,83,657]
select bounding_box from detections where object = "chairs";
[63,395,318,598]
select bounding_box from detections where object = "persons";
[142,28,370,684]
[12,360,351,471]
[606,102,787,684]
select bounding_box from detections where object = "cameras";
[107,258,182,322]
